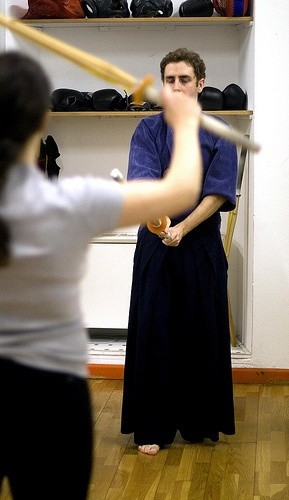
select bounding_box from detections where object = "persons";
[0,46,203,500]
[119,48,238,457]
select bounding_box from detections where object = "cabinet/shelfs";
[11,0,254,117]
